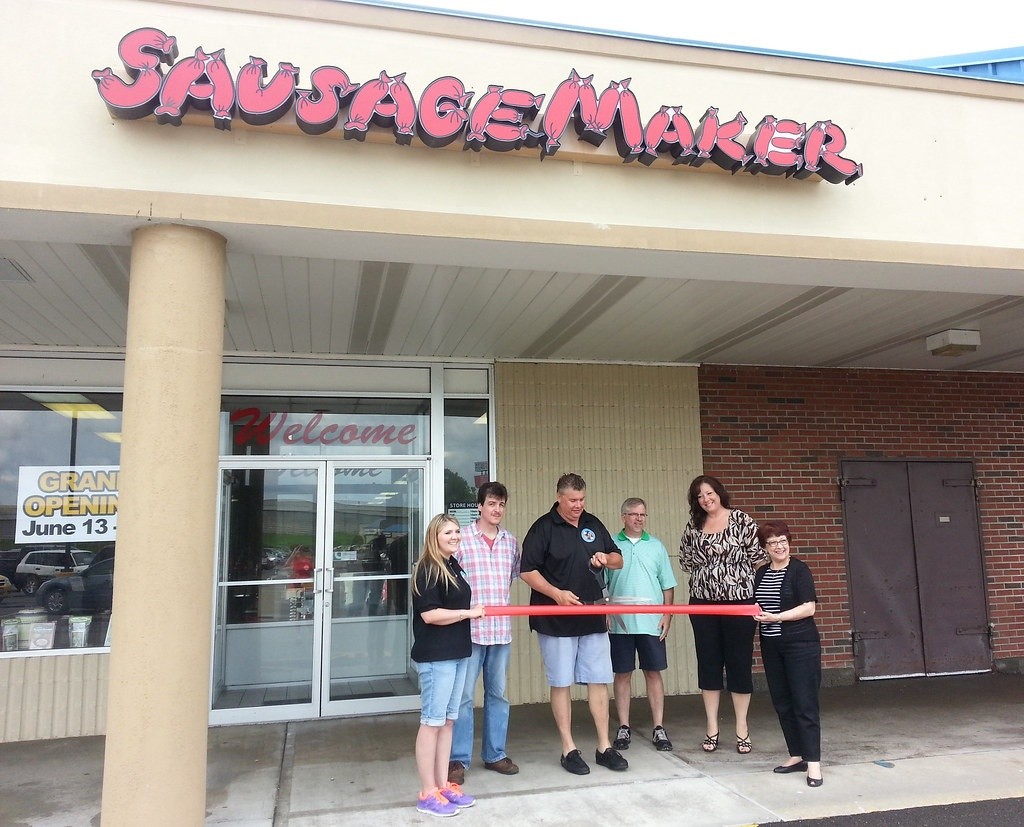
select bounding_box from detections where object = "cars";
[0,575,13,605]
[36,556,115,615]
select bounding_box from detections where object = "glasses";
[623,512,647,518]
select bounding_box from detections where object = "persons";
[346,519,392,615]
[520,473,630,776]
[447,480,519,784]
[753,518,825,788]
[604,497,677,751]
[288,544,314,587]
[678,475,769,754]
[409,513,476,816]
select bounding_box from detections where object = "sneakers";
[416,784,475,817]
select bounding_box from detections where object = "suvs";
[14,549,97,596]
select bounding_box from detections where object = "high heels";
[735,733,752,753]
[702,728,720,751]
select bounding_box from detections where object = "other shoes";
[485,757,521,775]
[651,726,671,751]
[448,760,465,784]
[595,748,629,769]
[560,750,590,775]
[613,726,631,749]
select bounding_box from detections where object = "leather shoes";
[806,775,825,786]
[774,758,808,773]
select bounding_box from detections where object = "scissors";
[573,558,652,635]
